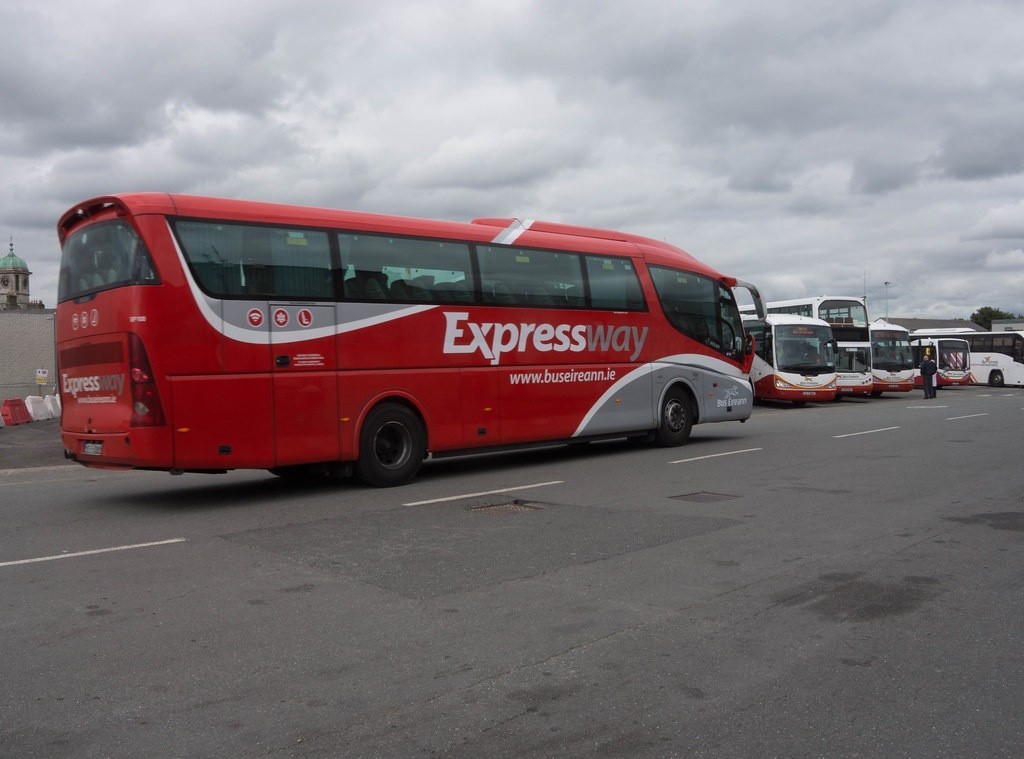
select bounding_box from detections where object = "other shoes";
[930,396,933,398]
[924,397,928,399]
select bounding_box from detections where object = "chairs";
[808,347,817,356]
[345,275,580,307]
[826,317,853,328]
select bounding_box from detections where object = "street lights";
[884,281,891,322]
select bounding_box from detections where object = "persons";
[920,356,938,399]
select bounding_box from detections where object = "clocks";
[0,276,11,288]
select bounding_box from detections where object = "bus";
[737,297,873,402]
[54,193,764,486]
[739,313,839,405]
[844,322,1024,395]
